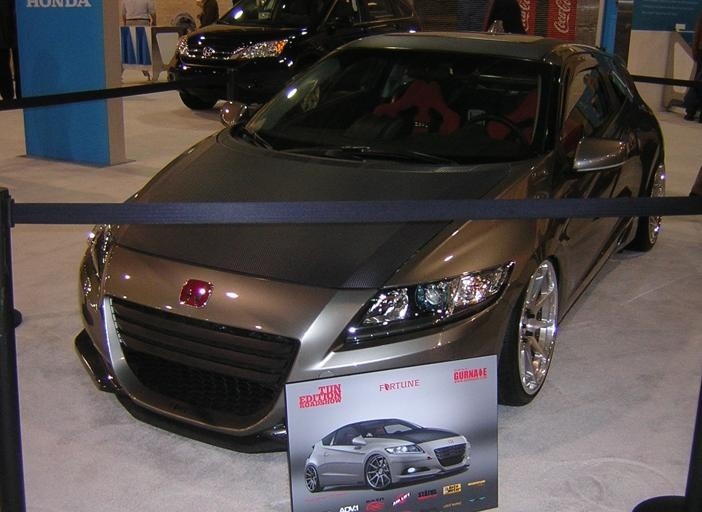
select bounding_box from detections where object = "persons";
[683,15,701,123]
[122,1,158,26]
[195,0,220,30]
[1,0,22,101]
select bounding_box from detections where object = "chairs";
[369,79,584,156]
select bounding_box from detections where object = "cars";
[302,417,473,496]
[73,32,667,454]
[167,0,424,110]
